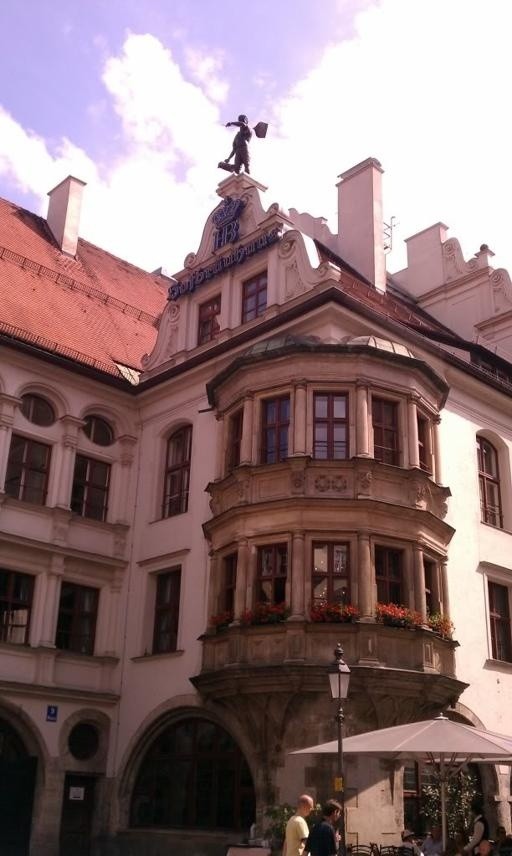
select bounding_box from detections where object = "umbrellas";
[289,710,512,856]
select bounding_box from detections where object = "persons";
[281,795,314,856]
[450,800,512,856]
[398,830,424,855]
[301,798,343,856]
[420,827,443,856]
[225,115,254,174]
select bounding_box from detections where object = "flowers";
[209,603,453,637]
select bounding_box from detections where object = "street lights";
[326,643,352,856]
